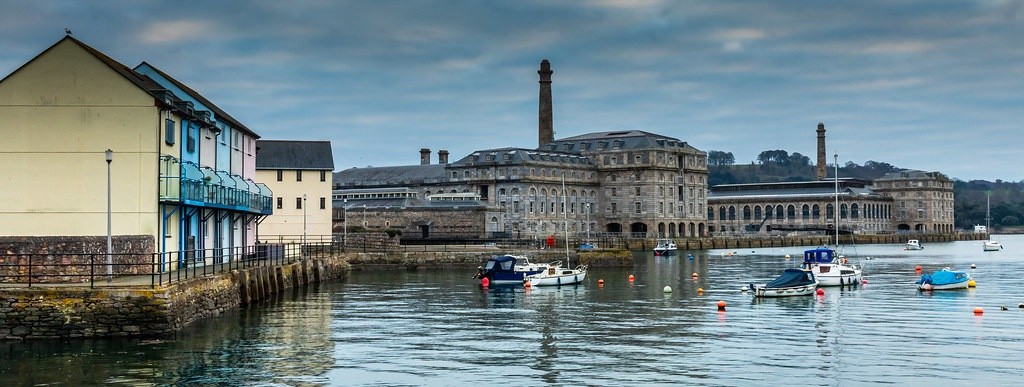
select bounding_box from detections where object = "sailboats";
[798,151,864,286]
[974,194,991,234]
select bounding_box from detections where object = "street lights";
[105,149,113,279]
[303,193,307,248]
[363,204,366,252]
[344,198,347,245]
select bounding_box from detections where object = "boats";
[916,266,976,290]
[750,221,820,297]
[906,239,924,250]
[470,172,588,287]
[982,240,1001,251]
[653,237,677,256]
